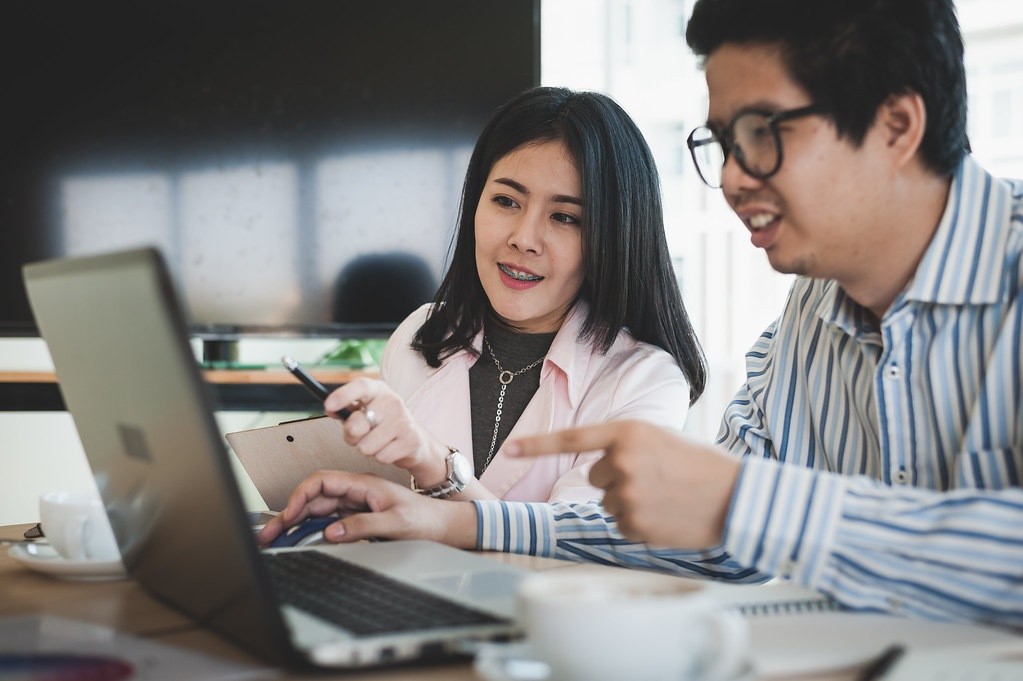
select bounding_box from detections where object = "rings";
[360,407,379,431]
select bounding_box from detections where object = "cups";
[514,568,750,681]
[38,492,120,560]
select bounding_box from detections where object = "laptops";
[20,248,540,675]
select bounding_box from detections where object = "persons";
[254,0,1022,635]
[323,85,708,506]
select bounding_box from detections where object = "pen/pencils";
[282,355,352,423]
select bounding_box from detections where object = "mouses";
[269,515,380,548]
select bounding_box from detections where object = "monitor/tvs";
[0,0,541,370]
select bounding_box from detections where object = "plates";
[8,541,127,582]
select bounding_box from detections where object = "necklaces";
[479,332,545,482]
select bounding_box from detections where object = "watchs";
[410,444,474,499]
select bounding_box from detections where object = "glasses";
[686,105,820,189]
